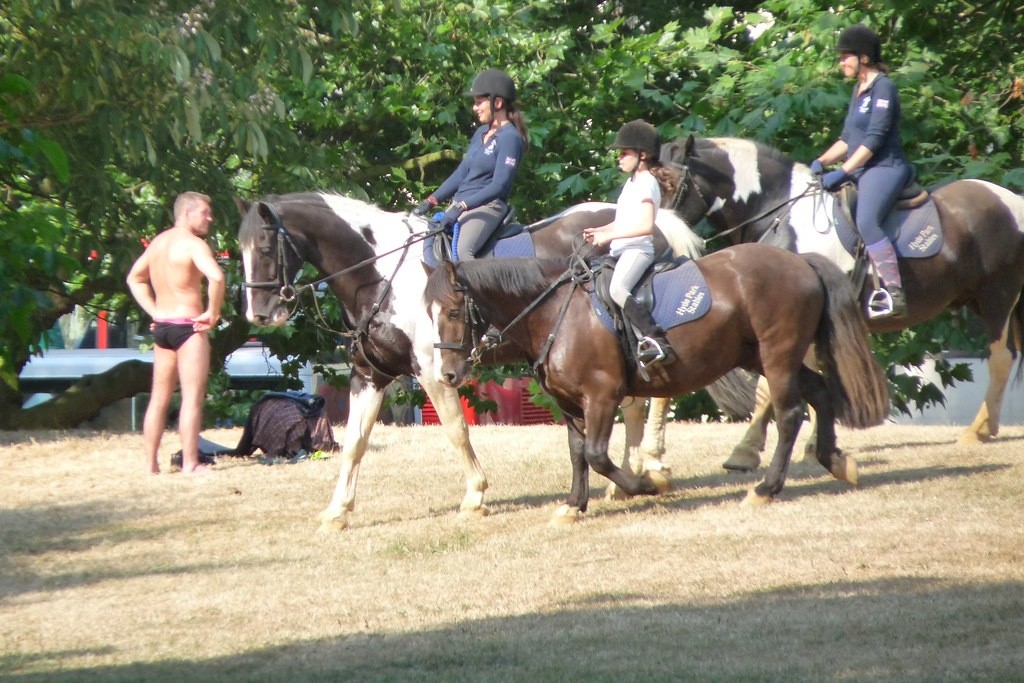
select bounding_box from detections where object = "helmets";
[462,69,515,104]
[607,119,660,159]
[829,23,880,61]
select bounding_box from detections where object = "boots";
[624,295,676,365]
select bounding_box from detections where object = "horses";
[229,185,708,538]
[654,134,1023,471]
[420,241,890,524]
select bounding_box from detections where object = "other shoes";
[479,324,503,347]
[869,284,908,320]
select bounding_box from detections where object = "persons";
[125,190,225,474]
[584,121,677,362]
[418,67,524,343]
[812,27,909,317]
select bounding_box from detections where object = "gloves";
[811,158,824,176]
[822,167,848,192]
[414,199,434,216]
[440,205,464,230]
